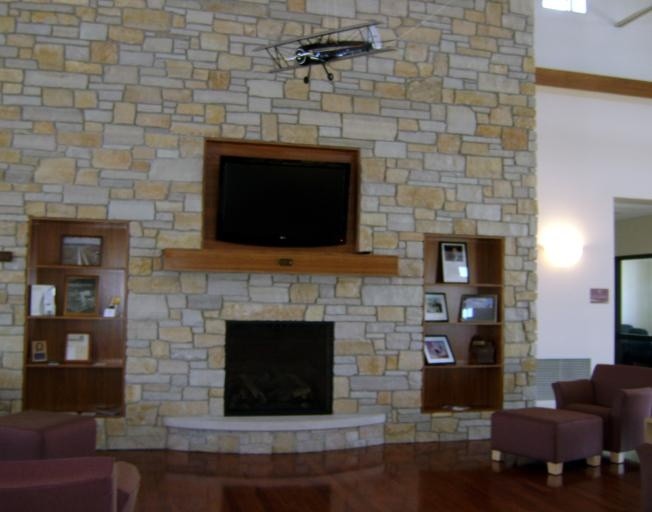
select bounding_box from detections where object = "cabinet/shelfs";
[22,216,131,420]
[422,232,507,414]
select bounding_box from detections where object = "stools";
[489,407,603,476]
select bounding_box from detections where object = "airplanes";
[254,17,398,84]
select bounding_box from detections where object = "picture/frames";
[424,333,457,365]
[424,292,449,323]
[29,337,50,364]
[58,233,104,267]
[438,240,471,285]
[61,330,94,365]
[458,292,499,325]
[62,273,100,320]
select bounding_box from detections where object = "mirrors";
[614,254,652,343]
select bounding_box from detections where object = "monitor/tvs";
[215,156,349,248]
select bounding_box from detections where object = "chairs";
[0,411,143,512]
[551,364,652,463]
[620,324,648,336]
